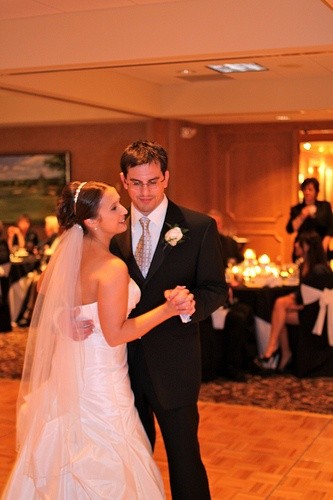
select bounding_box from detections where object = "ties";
[135,219,152,279]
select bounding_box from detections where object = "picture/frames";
[0,151,72,228]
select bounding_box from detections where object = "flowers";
[162,223,190,249]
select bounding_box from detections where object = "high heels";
[253,347,282,374]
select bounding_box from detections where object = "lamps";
[205,62,269,74]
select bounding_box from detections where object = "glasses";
[124,173,165,188]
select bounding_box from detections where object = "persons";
[10,182,194,499]
[208,180,333,379]
[1,186,75,333]
[67,139,226,499]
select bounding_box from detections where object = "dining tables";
[224,261,302,372]
